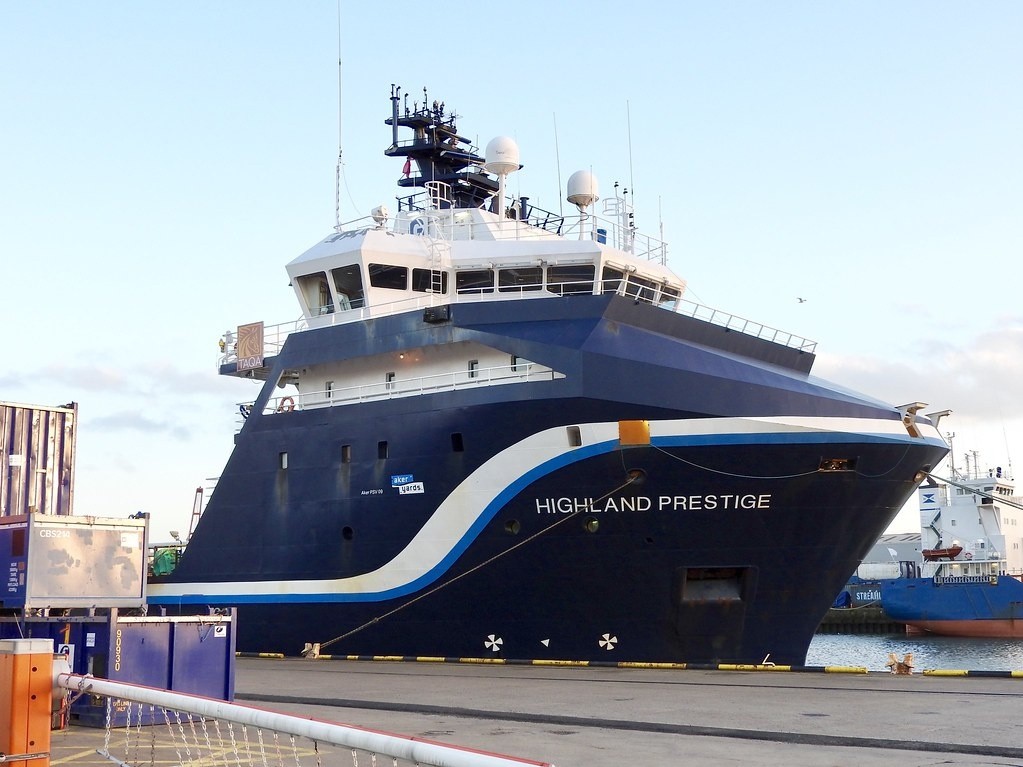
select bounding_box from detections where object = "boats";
[816,432,1023,642]
[142,26,956,674]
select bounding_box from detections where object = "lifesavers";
[280,397,295,412]
[965,553,972,559]
